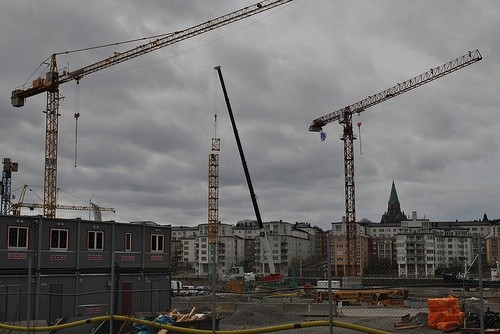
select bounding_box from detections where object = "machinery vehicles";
[304,289,411,307]
[213,65,289,289]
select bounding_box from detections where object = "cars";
[173,285,207,295]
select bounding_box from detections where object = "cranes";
[308,48,482,277]
[207,153,220,286]
[2,1,299,218]
